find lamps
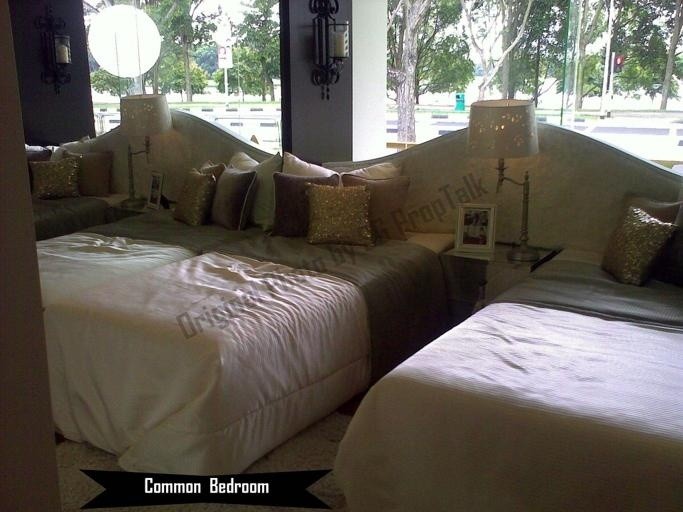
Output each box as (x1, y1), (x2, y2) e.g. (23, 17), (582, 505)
(467, 99), (540, 262)
(309, 0), (349, 100)
(120, 93), (172, 209)
(38, 3), (73, 97)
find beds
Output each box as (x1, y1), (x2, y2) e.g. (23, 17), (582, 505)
(43, 232), (457, 475)
(36, 208), (262, 310)
(32, 192), (128, 240)
(332, 248), (683, 512)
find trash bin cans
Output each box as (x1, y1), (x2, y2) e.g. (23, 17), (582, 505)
(456, 93), (464, 112)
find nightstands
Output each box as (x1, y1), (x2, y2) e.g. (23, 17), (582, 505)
(441, 242), (560, 326)
(105, 194), (151, 224)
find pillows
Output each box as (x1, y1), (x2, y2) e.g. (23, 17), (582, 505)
(601, 194), (683, 286)
(26, 138), (110, 199)
(269, 152), (410, 248)
(172, 151), (281, 233)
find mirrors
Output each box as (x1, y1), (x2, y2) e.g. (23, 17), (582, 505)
(8, 0), (293, 307)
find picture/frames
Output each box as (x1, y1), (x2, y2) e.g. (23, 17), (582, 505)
(146, 170), (164, 210)
(456, 202), (497, 256)
